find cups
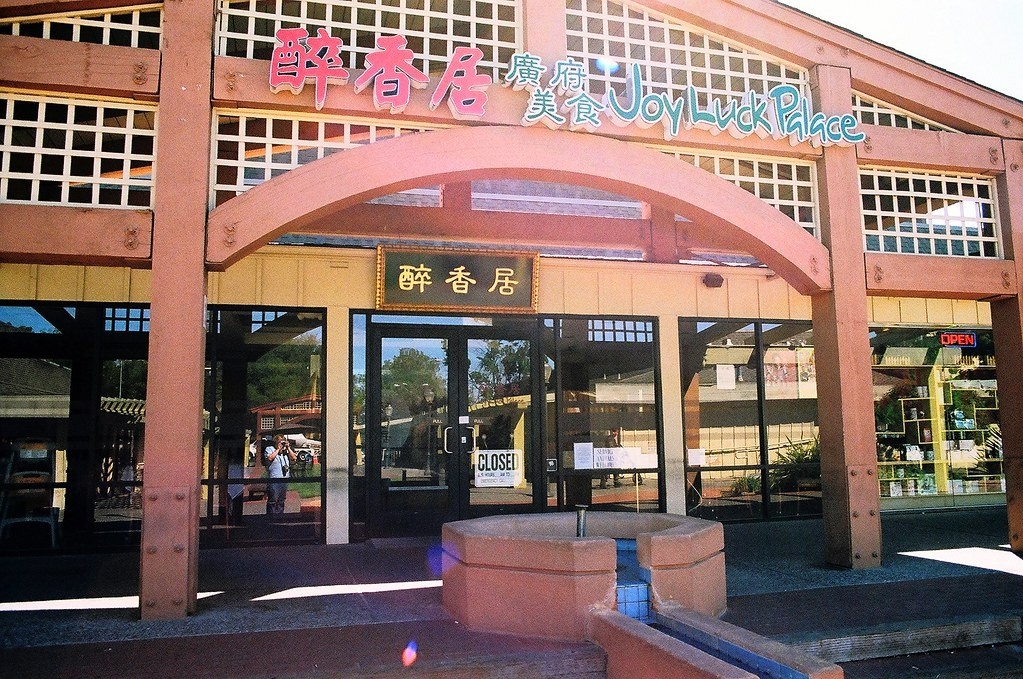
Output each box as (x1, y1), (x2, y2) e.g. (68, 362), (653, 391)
(911, 407), (918, 420)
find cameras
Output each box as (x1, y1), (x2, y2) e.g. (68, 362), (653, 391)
(281, 441), (286, 447)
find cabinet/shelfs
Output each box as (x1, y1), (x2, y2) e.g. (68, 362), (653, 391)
(871, 365), (1006, 498)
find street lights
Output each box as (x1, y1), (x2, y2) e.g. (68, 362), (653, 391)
(422, 387), (436, 476)
(384, 404), (394, 469)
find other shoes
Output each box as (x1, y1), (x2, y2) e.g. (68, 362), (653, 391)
(600, 485), (607, 489)
(615, 483), (621, 488)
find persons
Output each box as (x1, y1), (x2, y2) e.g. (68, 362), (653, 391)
(600, 426), (621, 488)
(264, 434), (297, 514)
